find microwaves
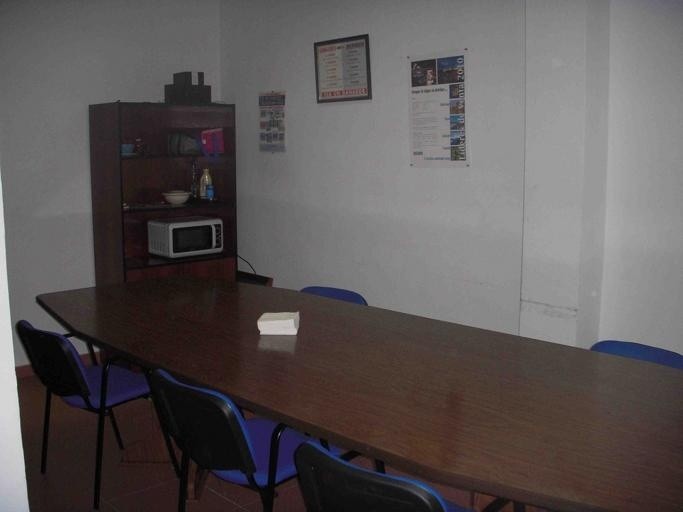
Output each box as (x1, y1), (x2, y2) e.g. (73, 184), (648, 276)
(148, 215), (223, 259)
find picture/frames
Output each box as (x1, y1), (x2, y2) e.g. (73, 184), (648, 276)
(313, 34), (372, 104)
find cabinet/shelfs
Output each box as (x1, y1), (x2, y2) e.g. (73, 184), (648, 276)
(88, 102), (238, 285)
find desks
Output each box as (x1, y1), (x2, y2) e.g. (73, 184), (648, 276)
(35, 272), (682, 511)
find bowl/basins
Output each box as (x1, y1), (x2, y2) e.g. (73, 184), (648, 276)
(161, 192), (192, 205)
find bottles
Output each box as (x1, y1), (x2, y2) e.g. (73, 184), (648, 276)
(190, 151), (214, 202)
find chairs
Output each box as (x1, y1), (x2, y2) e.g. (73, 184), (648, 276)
(146, 370), (389, 510)
(290, 440), (478, 512)
(16, 319), (182, 508)
